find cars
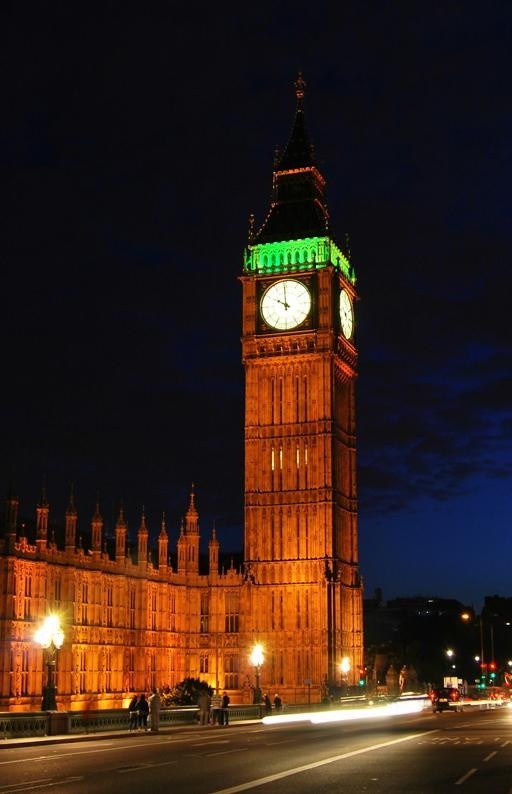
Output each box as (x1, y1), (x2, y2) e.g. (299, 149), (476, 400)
(430, 687), (464, 713)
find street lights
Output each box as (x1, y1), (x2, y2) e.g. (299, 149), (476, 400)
(33, 614), (65, 711)
(341, 657), (350, 697)
(250, 644), (264, 703)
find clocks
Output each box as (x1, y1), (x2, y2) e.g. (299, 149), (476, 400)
(259, 277), (312, 332)
(339, 287), (355, 342)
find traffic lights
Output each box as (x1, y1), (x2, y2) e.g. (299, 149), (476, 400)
(477, 663), (495, 689)
(358, 669), (366, 688)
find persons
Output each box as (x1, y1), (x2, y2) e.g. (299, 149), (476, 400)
(211, 693), (224, 725)
(221, 690), (229, 727)
(149, 687), (161, 731)
(264, 693), (272, 713)
(136, 694), (150, 732)
(128, 695), (139, 732)
(198, 690), (210, 727)
(273, 693), (283, 712)
